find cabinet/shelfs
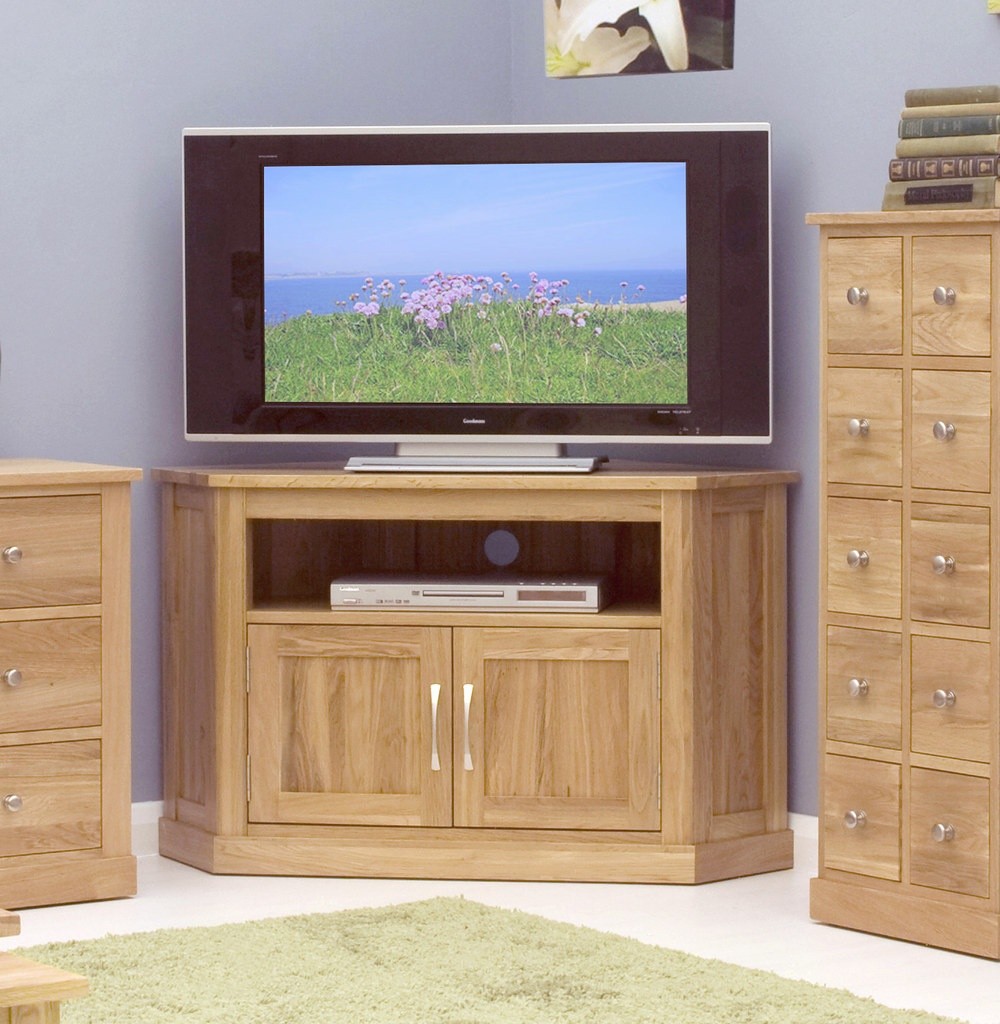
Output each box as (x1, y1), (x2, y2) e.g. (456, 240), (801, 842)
(2, 452), (145, 910)
(801, 208), (1000, 962)
(154, 464), (795, 889)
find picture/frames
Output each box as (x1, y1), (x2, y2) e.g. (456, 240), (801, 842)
(541, 0), (736, 83)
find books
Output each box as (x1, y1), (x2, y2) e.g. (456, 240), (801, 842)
(876, 85), (1000, 211)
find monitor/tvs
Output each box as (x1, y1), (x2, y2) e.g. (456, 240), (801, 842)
(180, 121), (772, 476)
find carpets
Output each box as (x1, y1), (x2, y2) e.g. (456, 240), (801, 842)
(0, 888), (976, 1023)
